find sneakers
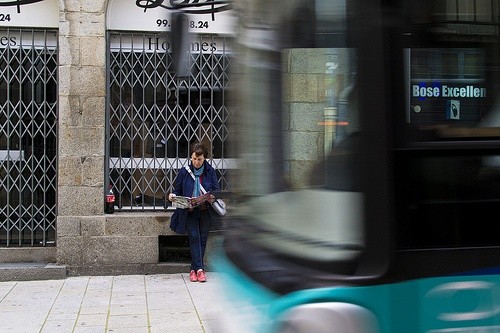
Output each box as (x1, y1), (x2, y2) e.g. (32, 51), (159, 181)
(190, 270), (197, 282)
(196, 270), (206, 281)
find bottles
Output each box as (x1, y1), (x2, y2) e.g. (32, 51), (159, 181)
(105, 185), (115, 214)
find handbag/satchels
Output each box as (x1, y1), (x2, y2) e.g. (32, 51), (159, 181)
(212, 198), (226, 216)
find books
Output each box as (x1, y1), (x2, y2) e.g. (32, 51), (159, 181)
(170, 191), (211, 210)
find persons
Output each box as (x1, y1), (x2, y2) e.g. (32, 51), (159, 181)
(168, 144), (220, 283)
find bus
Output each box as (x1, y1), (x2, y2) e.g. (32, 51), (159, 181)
(170, 1), (500, 333)
